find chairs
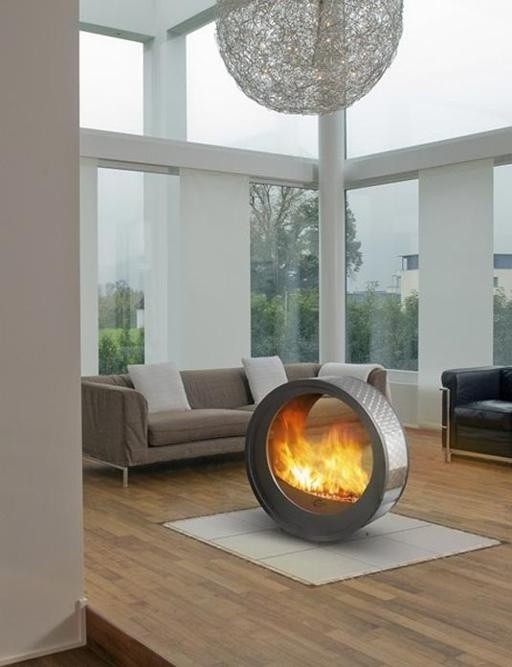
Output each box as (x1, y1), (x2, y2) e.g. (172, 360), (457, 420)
(442, 367), (511, 467)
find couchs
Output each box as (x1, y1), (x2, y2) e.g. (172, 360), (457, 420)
(81, 363), (389, 487)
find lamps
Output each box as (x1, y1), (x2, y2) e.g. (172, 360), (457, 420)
(217, 1), (404, 113)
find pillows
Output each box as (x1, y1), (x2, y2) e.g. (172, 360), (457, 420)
(242, 355), (288, 405)
(130, 364), (191, 414)
(154, 505), (508, 587)
